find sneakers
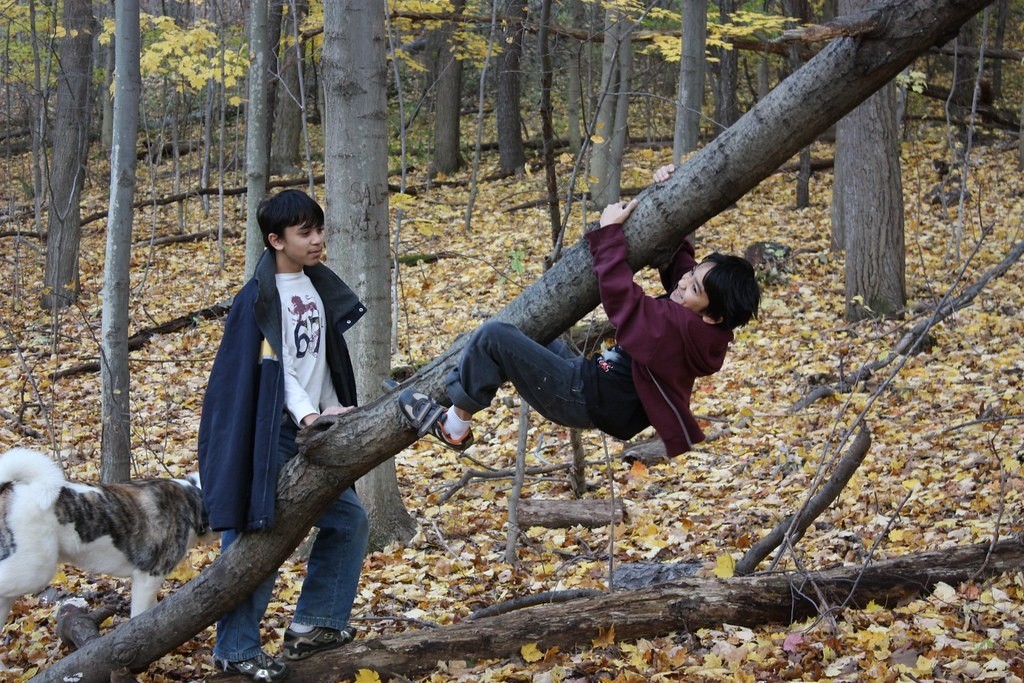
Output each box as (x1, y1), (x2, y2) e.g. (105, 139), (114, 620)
(215, 653), (290, 683)
(284, 623), (356, 661)
(398, 389), (474, 451)
(381, 378), (401, 395)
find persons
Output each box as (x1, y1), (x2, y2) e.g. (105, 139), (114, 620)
(379, 165), (761, 451)
(197, 189), (371, 683)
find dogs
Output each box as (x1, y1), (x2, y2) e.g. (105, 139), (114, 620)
(0, 449), (228, 628)
